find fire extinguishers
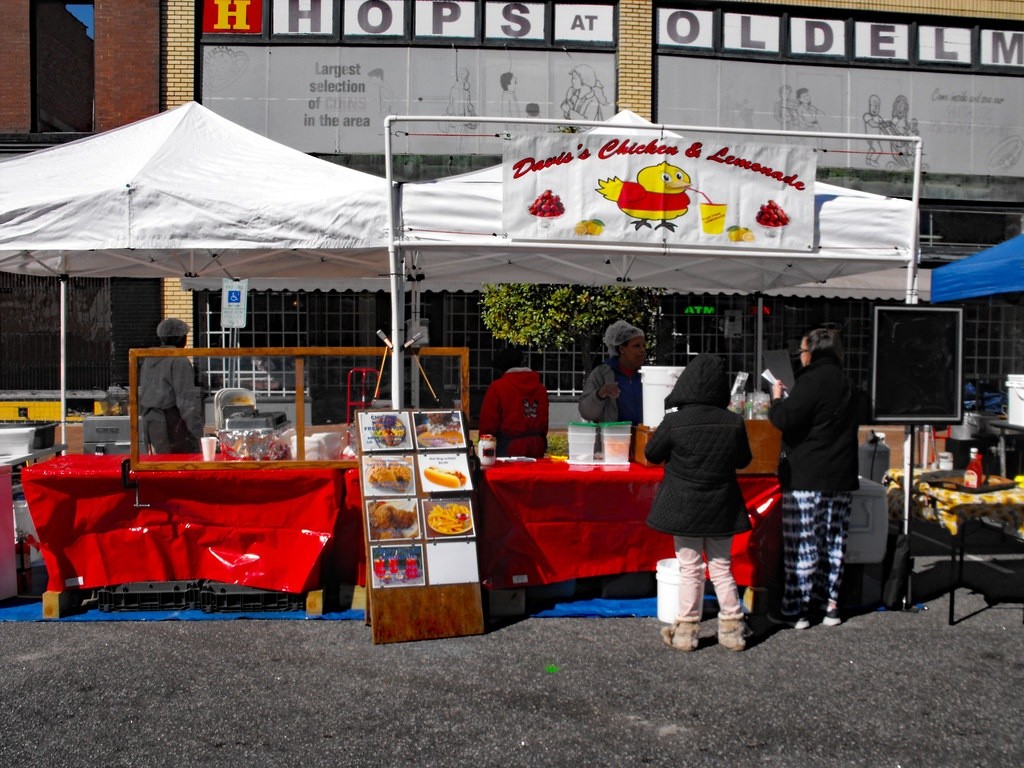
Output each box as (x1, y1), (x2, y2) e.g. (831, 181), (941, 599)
(14, 527), (40, 595)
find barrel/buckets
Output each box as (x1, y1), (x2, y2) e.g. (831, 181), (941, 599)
(939, 452), (953, 471)
(1005, 374), (1024, 427)
(656, 558), (706, 624)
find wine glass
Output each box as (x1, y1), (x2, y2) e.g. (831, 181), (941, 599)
(374, 556), (387, 586)
(405, 553), (417, 584)
(388, 555), (400, 583)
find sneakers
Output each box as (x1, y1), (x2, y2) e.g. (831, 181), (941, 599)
(809, 603), (842, 627)
(766, 609), (810, 629)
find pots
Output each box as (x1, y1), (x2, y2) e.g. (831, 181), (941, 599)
(950, 412), (981, 440)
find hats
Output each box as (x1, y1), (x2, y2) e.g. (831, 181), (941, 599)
(490, 347), (522, 372)
(157, 318), (189, 337)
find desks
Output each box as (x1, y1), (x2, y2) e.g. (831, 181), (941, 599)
(1, 442), (787, 619)
(882, 466), (1024, 626)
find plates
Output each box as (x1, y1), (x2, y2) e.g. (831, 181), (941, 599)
(428, 518), (472, 535)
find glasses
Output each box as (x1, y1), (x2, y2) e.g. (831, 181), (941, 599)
(798, 349), (809, 353)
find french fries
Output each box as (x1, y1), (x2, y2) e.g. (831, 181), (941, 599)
(429, 502), (472, 532)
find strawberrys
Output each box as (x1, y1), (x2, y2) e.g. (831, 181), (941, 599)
(756, 200), (789, 226)
(529, 190), (565, 217)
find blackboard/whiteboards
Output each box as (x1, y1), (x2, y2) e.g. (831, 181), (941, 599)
(867, 301), (965, 426)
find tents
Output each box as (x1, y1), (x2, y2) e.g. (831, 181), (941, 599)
(929, 233), (1024, 304)
(0, 107), (919, 607)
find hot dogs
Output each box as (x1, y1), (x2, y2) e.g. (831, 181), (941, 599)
(418, 430), (464, 446)
(424, 467), (466, 488)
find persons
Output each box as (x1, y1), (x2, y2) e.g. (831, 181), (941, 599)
(769, 328), (859, 629)
(578, 320), (649, 425)
(138, 319), (215, 453)
(475, 347), (550, 460)
(645, 356), (751, 652)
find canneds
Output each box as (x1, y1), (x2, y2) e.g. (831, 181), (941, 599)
(477, 435), (496, 465)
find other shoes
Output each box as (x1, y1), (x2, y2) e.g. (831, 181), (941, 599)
(717, 619), (754, 651)
(661, 620), (701, 651)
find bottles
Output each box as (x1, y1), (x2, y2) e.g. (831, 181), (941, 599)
(477, 435), (497, 465)
(963, 447), (983, 489)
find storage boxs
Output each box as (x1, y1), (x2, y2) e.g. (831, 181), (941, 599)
(566, 420), (635, 471)
(636, 424), (665, 466)
(1004, 373), (1024, 427)
(735, 419), (782, 475)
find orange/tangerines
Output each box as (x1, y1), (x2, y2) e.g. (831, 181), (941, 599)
(575, 220), (603, 235)
(728, 227), (755, 242)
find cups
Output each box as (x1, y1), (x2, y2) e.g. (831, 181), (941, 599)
(730, 390), (770, 420)
(200, 437), (217, 461)
